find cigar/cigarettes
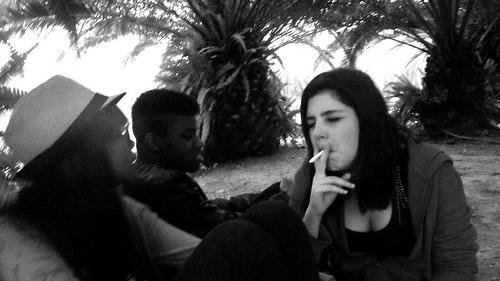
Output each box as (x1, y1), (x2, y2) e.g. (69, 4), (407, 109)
(309, 150), (325, 163)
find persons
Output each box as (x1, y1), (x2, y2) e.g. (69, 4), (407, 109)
(290, 69), (479, 281)
(120, 89), (297, 238)
(0, 76), (320, 281)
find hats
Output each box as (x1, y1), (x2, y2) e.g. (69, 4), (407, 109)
(3, 75), (126, 181)
